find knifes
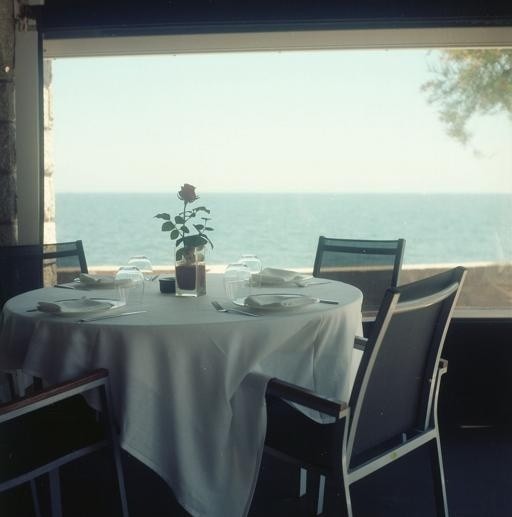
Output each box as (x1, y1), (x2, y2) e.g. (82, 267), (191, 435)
(54, 285), (90, 291)
(78, 310), (147, 323)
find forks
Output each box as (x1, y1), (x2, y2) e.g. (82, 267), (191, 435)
(211, 301), (261, 317)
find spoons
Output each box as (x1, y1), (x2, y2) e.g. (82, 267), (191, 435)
(294, 281), (330, 287)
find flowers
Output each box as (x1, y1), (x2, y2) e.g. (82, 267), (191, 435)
(152, 183), (215, 266)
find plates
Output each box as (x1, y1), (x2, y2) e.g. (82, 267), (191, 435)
(233, 293), (320, 312)
(50, 298), (126, 318)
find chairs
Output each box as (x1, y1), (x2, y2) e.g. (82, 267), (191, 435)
(0, 368), (129, 517)
(262, 265), (469, 517)
(313, 236), (406, 338)
(0, 240), (88, 401)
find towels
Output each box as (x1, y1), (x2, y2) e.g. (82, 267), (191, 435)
(80, 273), (115, 284)
(256, 267), (300, 284)
(244, 293), (309, 307)
(38, 295), (114, 314)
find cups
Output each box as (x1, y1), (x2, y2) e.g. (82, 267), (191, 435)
(223, 254), (263, 299)
(114, 255), (152, 317)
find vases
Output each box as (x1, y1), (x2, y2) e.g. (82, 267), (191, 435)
(175, 246), (206, 297)
